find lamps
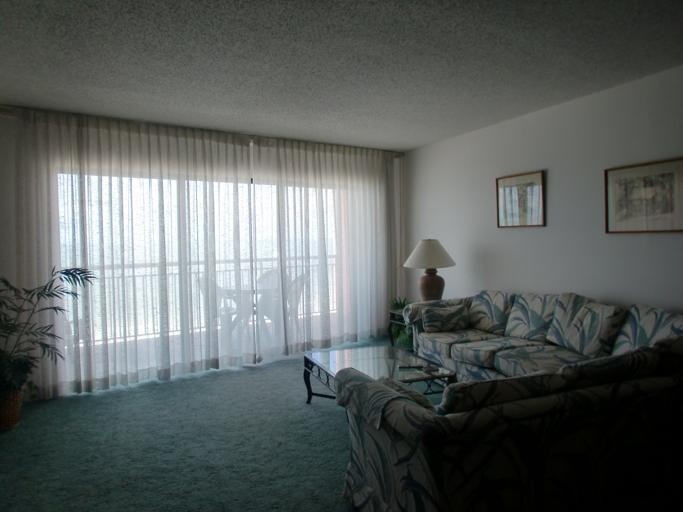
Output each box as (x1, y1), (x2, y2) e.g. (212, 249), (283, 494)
(401, 239), (455, 301)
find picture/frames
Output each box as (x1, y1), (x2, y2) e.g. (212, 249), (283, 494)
(604, 156), (683, 234)
(495, 169), (546, 228)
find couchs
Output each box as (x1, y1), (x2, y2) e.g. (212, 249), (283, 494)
(333, 289), (682, 512)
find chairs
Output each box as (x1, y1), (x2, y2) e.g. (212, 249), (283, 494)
(191, 262), (312, 354)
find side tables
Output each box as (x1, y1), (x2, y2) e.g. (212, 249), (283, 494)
(386, 309), (406, 346)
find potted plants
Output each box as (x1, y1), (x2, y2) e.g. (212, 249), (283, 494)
(0, 265), (99, 430)
